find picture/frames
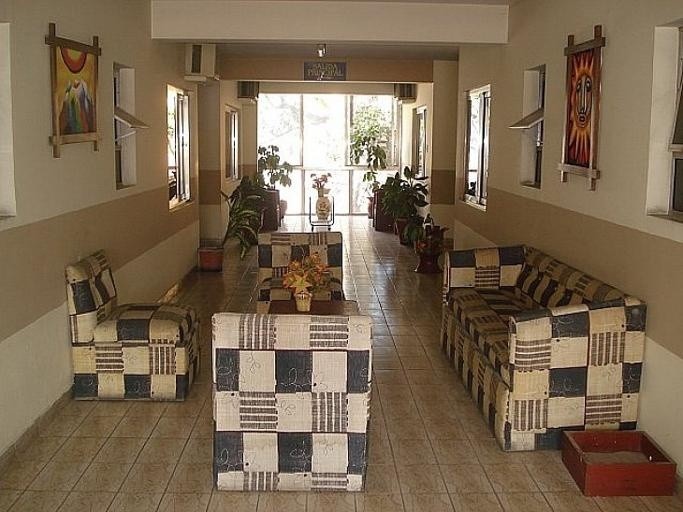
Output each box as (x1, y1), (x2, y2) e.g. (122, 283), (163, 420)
(558, 25), (605, 191)
(44, 23), (102, 158)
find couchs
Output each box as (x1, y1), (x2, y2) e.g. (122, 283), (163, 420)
(440, 244), (646, 454)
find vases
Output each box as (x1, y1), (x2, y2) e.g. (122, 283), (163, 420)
(294, 293), (312, 312)
(316, 197), (331, 219)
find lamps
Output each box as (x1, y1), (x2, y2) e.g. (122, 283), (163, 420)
(317, 44), (327, 58)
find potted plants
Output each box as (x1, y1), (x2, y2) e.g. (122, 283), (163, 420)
(403, 214), (450, 274)
(349, 104), (386, 218)
(198, 176), (269, 271)
(258, 144), (293, 218)
(382, 166), (429, 246)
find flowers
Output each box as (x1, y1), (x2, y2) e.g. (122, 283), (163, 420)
(311, 173), (332, 197)
(283, 252), (328, 293)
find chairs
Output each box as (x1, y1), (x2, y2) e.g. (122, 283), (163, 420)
(211, 312), (373, 493)
(65, 249), (200, 402)
(258, 233), (343, 315)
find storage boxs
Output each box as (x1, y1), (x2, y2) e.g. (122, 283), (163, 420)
(562, 429), (677, 496)
